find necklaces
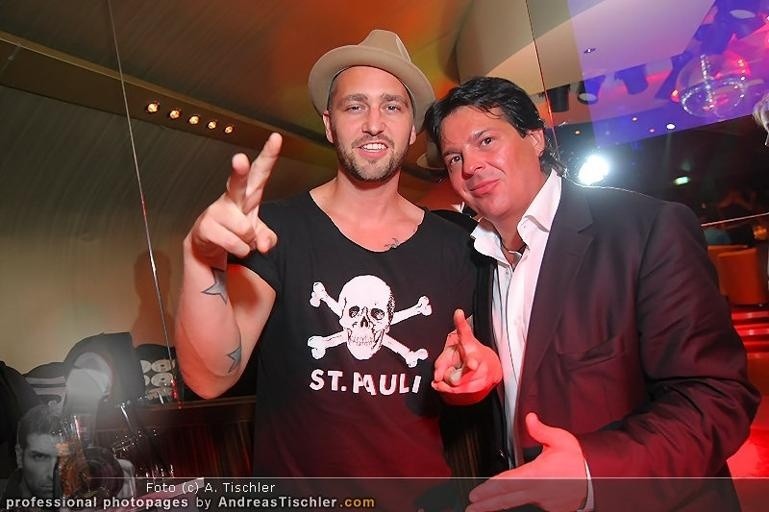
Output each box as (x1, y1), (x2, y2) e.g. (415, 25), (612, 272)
(500, 238), (527, 263)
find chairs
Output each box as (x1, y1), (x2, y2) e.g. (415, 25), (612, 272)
(707, 244), (769, 310)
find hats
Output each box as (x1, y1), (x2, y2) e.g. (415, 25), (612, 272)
(308, 30), (434, 135)
(417, 139), (446, 172)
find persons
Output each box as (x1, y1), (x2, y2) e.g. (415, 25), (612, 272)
(0, 403), (71, 512)
(172, 28), (503, 511)
(421, 74), (763, 512)
